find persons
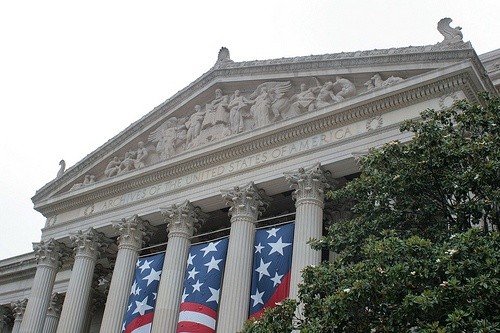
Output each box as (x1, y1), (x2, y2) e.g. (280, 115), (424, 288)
(63, 65), (401, 189)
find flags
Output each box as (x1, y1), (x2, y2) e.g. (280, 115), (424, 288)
(175, 238), (229, 333)
(121, 253), (165, 333)
(248, 223), (293, 319)
